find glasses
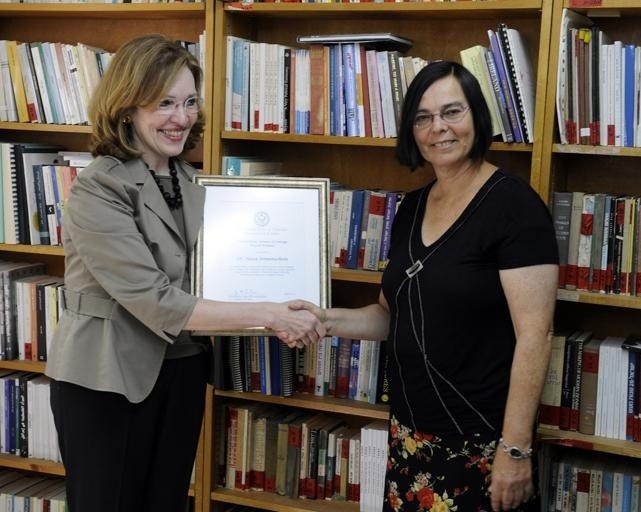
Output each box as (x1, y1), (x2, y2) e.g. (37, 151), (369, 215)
(155, 95), (205, 115)
(414, 104), (470, 130)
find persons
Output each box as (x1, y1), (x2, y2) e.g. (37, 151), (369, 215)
(47, 33), (327, 512)
(264, 61), (560, 512)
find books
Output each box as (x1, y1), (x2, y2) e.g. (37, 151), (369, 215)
(230, 335), (389, 406)
(221, 155), (405, 273)
(0, 467), (68, 512)
(555, 9), (641, 148)
(0, 142), (94, 246)
(226, 24), (535, 143)
(550, 453), (641, 512)
(0, 40), (116, 127)
(538, 330), (641, 441)
(552, 191), (641, 298)
(0, 368), (63, 463)
(219, 402), (389, 512)
(0, 261), (66, 362)
(176, 30), (205, 103)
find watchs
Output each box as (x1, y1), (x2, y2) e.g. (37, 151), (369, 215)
(500, 438), (533, 460)
